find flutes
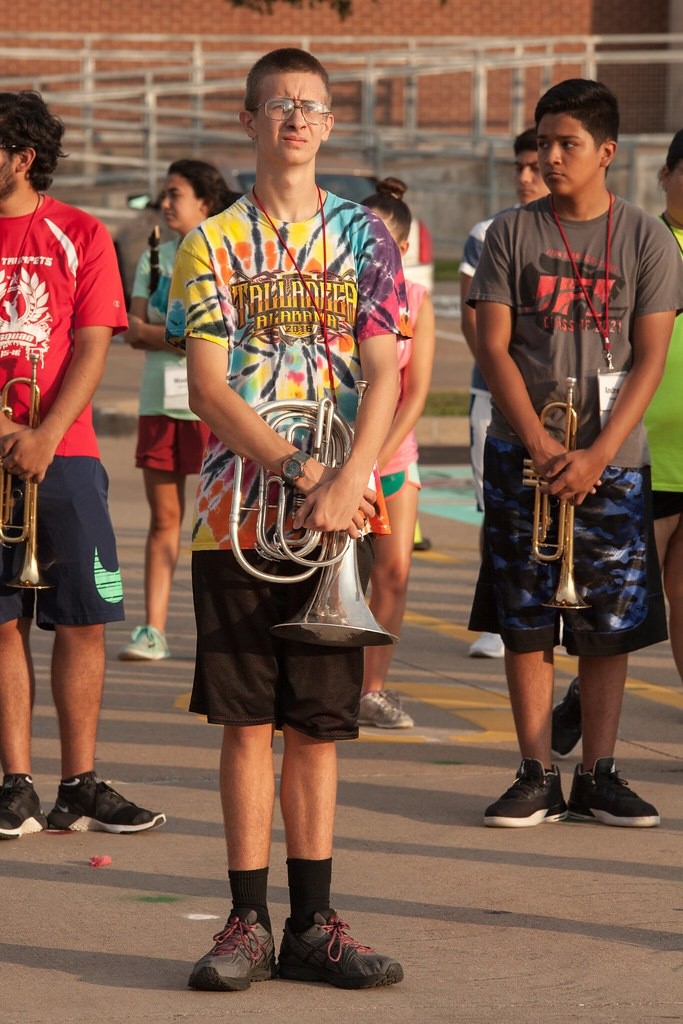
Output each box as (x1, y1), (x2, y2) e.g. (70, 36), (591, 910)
(148, 224), (160, 294)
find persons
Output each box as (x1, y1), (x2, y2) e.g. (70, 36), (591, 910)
(0, 90), (166, 839)
(119, 158), (235, 661)
(350, 178), (435, 729)
(459, 78), (683, 826)
(164, 46), (412, 988)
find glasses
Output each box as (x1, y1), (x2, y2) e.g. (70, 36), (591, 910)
(253, 98), (331, 125)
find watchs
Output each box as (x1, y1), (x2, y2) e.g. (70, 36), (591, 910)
(278, 450), (312, 487)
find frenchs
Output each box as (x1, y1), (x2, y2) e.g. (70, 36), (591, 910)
(220, 379), (402, 652)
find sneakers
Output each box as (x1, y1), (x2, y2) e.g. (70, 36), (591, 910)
(277, 907), (404, 989)
(484, 758), (569, 827)
(567, 755), (660, 827)
(357, 690), (415, 728)
(47, 777), (166, 834)
(469, 633), (505, 658)
(117, 625), (168, 661)
(0, 775), (48, 837)
(188, 908), (276, 991)
(550, 676), (584, 758)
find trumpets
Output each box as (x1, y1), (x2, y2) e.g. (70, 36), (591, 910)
(519, 373), (593, 612)
(0, 351), (53, 594)
